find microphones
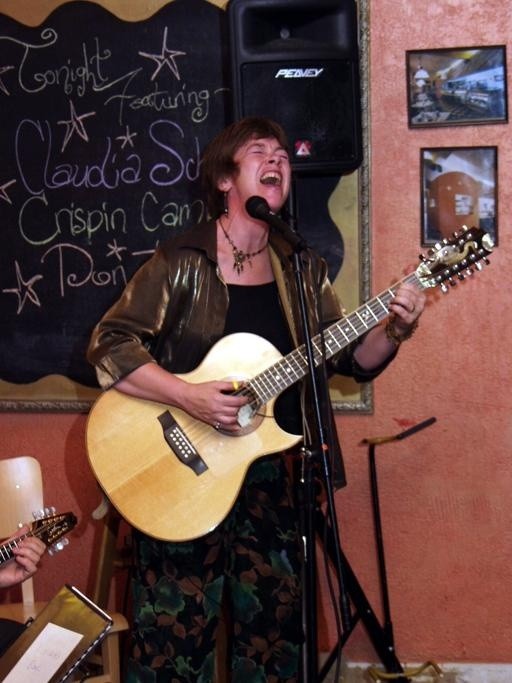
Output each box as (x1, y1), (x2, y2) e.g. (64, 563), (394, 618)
(245, 196), (308, 252)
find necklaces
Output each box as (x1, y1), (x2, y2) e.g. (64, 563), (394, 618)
(218, 217), (269, 275)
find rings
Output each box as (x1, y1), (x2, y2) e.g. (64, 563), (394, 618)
(411, 304), (415, 313)
(215, 421), (221, 429)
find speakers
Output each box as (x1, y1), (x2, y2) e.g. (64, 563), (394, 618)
(225, 0), (364, 175)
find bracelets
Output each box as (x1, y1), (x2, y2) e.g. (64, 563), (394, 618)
(385, 313), (419, 344)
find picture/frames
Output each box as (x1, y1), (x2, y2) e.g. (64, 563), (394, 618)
(405, 45), (509, 250)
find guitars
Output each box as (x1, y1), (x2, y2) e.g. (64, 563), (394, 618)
(0, 506), (77, 564)
(85, 226), (497, 547)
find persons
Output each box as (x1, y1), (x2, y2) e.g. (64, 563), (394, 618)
(0, 526), (47, 589)
(85, 116), (426, 683)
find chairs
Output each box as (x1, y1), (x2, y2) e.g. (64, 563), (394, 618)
(2, 454), (130, 682)
